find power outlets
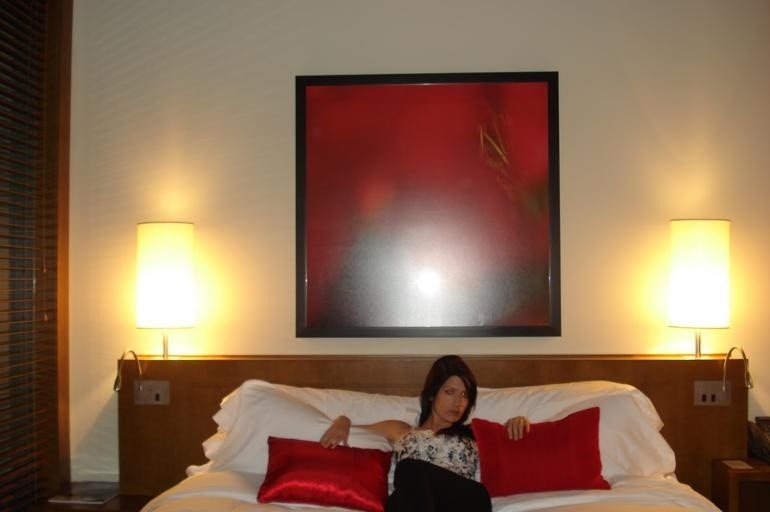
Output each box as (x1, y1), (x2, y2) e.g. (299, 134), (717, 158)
(693, 381), (730, 405)
(134, 380), (169, 405)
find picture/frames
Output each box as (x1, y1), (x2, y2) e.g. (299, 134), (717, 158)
(296, 71), (562, 336)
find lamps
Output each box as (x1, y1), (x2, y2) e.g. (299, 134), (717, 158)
(669, 217), (732, 357)
(137, 222), (198, 359)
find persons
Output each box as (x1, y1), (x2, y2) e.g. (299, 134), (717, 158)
(319, 355), (530, 511)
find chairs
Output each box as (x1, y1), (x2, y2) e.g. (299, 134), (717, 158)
(750, 416), (770, 460)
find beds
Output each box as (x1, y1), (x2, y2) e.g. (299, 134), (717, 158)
(112, 355), (751, 511)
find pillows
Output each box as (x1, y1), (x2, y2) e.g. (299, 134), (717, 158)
(257, 437), (394, 510)
(473, 406), (613, 495)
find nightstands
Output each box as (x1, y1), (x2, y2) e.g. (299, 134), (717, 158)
(711, 459), (769, 511)
